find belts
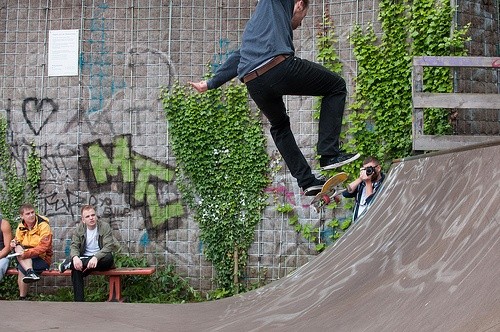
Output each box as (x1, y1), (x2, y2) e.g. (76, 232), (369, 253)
(244, 54), (290, 85)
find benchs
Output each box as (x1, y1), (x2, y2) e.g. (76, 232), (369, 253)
(6, 267), (156, 302)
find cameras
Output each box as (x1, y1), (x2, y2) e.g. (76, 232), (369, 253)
(364, 166), (378, 183)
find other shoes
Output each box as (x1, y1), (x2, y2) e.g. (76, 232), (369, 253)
(303, 179), (326, 196)
(58, 259), (73, 273)
(320, 141), (361, 171)
(22, 268), (40, 284)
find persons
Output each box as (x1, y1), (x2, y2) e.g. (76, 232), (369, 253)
(9, 204), (53, 299)
(190, 0), (361, 196)
(59, 205), (116, 302)
(341, 156), (386, 223)
(0, 216), (13, 285)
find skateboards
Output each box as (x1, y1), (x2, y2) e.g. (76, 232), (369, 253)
(311, 172), (349, 214)
